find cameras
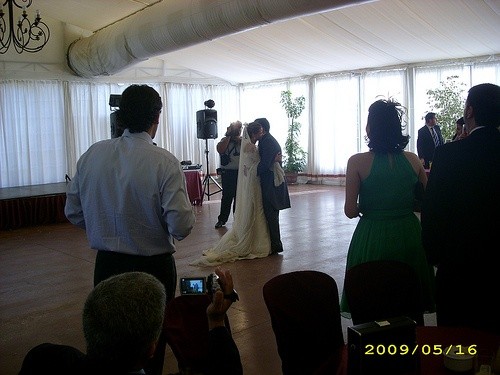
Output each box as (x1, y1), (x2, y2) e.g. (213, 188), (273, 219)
(180, 273), (223, 302)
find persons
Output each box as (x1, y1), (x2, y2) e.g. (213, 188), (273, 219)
(213, 121), (241, 230)
(64, 85), (194, 375)
(422, 83), (500, 334)
(452, 116), (468, 142)
(18, 268), (245, 375)
(199, 121), (285, 264)
(417, 112), (444, 168)
(340, 100), (431, 323)
(244, 118), (291, 258)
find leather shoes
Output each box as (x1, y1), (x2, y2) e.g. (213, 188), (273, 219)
(215, 221), (226, 228)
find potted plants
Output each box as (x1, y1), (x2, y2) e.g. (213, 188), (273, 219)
(279, 89), (310, 184)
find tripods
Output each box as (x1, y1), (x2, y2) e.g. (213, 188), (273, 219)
(201, 139), (222, 201)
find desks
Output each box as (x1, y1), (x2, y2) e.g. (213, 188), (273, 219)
(334, 325), (500, 375)
(182, 169), (202, 206)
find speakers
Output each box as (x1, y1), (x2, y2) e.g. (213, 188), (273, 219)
(196, 110), (217, 140)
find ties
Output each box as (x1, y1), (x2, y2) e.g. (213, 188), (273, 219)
(430, 128), (440, 147)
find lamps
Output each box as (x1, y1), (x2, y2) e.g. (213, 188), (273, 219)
(0, 0), (51, 54)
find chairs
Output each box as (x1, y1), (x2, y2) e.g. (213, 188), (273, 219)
(162, 294), (244, 375)
(262, 270), (344, 375)
(344, 260), (434, 326)
